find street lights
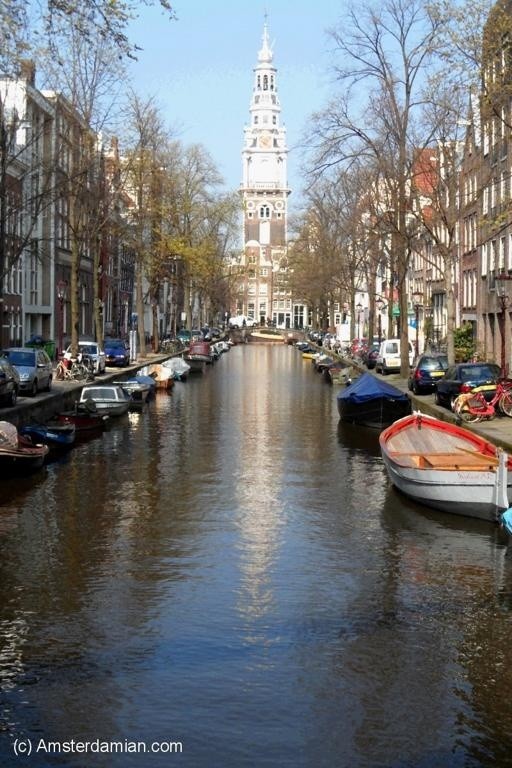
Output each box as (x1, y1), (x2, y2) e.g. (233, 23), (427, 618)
(489, 271), (512, 366)
(55, 277), (69, 359)
(376, 297), (385, 340)
(356, 301), (365, 338)
(167, 249), (182, 336)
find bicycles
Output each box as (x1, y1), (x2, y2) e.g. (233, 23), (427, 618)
(54, 345), (95, 383)
(449, 375), (512, 424)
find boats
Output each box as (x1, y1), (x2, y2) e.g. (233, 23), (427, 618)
(335, 370), (415, 438)
(134, 361), (176, 386)
(283, 337), (355, 386)
(186, 337), (235, 366)
(376, 408), (512, 524)
(160, 355), (191, 382)
(501, 506), (512, 539)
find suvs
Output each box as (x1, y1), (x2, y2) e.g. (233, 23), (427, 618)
(102, 337), (130, 352)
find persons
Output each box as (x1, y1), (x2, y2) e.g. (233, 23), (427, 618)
(242, 318), (246, 327)
(267, 315), (272, 326)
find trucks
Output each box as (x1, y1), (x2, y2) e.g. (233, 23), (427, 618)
(228, 314), (258, 329)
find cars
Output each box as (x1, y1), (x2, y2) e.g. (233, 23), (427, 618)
(103, 346), (130, 367)
(431, 359), (506, 413)
(302, 323), (388, 366)
(3, 345), (54, 398)
(159, 324), (222, 351)
(0, 347), (21, 409)
(59, 340), (107, 375)
(0, 374), (158, 471)
(406, 350), (450, 396)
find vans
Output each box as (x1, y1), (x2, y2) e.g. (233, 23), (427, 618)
(374, 336), (416, 375)
